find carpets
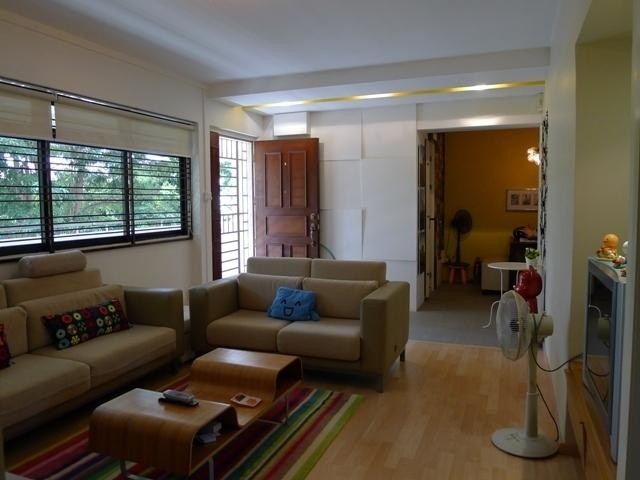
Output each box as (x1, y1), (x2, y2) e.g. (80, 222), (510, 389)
(5, 369), (363, 480)
(408, 282), (542, 351)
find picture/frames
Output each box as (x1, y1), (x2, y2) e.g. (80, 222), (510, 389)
(505, 188), (538, 213)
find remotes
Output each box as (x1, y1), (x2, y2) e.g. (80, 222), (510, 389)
(159, 398), (199, 406)
(164, 389), (194, 402)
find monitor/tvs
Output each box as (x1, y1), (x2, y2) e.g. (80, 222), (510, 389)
(582, 258), (627, 462)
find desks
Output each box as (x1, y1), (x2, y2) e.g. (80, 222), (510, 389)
(483, 262), (537, 329)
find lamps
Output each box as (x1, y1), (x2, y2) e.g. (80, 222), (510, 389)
(51, 100), (57, 140)
(527, 146), (542, 167)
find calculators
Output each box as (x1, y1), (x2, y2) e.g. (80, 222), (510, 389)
(230, 392), (263, 406)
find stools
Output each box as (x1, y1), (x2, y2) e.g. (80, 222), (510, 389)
(448, 266), (468, 289)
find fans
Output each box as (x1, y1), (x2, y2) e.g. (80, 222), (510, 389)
(490, 290), (560, 459)
(587, 303), (612, 349)
(449, 209), (472, 268)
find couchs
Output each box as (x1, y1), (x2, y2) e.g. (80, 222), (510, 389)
(0, 268), (185, 442)
(187, 255), (410, 393)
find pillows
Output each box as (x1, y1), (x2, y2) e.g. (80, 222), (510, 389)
(302, 277), (379, 320)
(16, 284), (128, 352)
(39, 297), (132, 351)
(0, 324), (15, 369)
(267, 287), (321, 321)
(238, 273), (302, 312)
(0, 306), (29, 358)
(17, 250), (87, 278)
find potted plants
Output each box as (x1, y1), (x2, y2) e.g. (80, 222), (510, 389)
(525, 248), (540, 269)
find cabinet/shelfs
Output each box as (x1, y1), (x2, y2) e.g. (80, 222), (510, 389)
(509, 242), (537, 291)
(481, 257), (510, 292)
